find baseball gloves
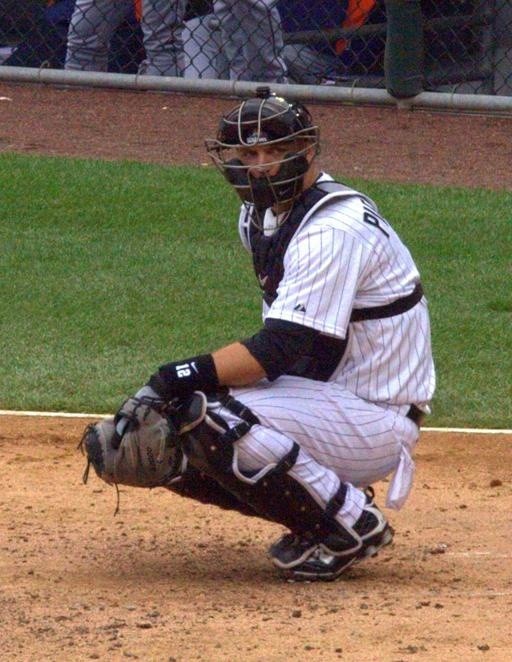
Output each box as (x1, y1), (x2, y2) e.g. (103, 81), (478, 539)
(77, 397), (182, 515)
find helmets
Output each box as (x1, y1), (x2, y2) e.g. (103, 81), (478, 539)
(203, 85), (321, 213)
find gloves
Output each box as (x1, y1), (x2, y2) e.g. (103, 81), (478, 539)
(109, 374), (171, 451)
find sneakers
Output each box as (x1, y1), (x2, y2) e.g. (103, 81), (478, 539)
(269, 485), (396, 580)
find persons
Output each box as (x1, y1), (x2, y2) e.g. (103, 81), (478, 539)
(64, 0), (184, 78)
(180, 1), (292, 85)
(70, 85), (437, 583)
